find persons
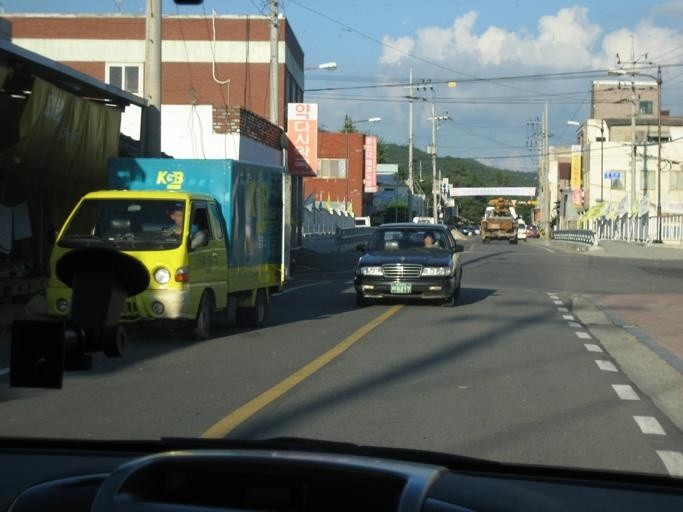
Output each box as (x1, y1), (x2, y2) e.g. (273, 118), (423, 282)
(423, 231), (443, 249)
(159, 204), (207, 253)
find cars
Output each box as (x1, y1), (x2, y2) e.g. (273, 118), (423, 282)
(458, 225), (479, 235)
(351, 221), (465, 308)
(525, 225), (541, 239)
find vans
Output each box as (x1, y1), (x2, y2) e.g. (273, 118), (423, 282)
(516, 219), (528, 242)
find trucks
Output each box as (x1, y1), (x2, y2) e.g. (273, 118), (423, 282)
(479, 198), (520, 245)
(40, 151), (294, 342)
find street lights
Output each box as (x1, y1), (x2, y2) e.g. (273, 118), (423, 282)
(567, 117), (606, 205)
(345, 116), (383, 213)
(605, 64), (665, 244)
